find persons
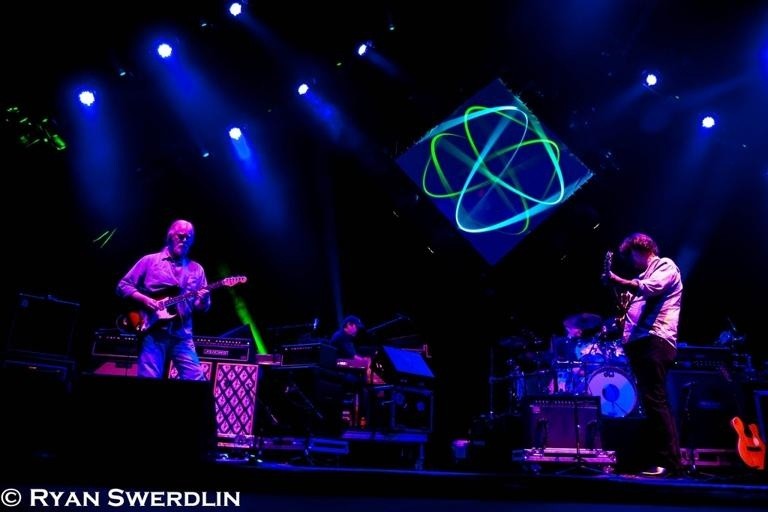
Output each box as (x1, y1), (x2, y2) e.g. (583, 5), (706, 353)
(549, 318), (584, 362)
(320, 316), (372, 373)
(113, 218), (214, 382)
(600, 233), (685, 477)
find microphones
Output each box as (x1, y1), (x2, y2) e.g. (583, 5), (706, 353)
(536, 414), (549, 426)
(313, 318), (317, 329)
(586, 421), (599, 430)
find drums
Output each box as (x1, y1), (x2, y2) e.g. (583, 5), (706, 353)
(586, 369), (638, 419)
(549, 362), (586, 394)
(576, 338), (603, 364)
(606, 339), (630, 366)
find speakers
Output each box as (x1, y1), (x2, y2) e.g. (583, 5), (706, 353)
(69, 374), (210, 488)
(522, 395), (604, 449)
(253, 366), (342, 437)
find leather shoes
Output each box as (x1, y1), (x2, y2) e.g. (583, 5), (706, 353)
(640, 464), (683, 477)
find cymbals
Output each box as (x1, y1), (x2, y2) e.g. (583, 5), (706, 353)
(564, 314), (602, 330)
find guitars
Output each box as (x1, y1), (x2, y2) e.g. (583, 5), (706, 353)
(731, 368), (765, 471)
(122, 275), (248, 333)
(602, 251), (633, 327)
